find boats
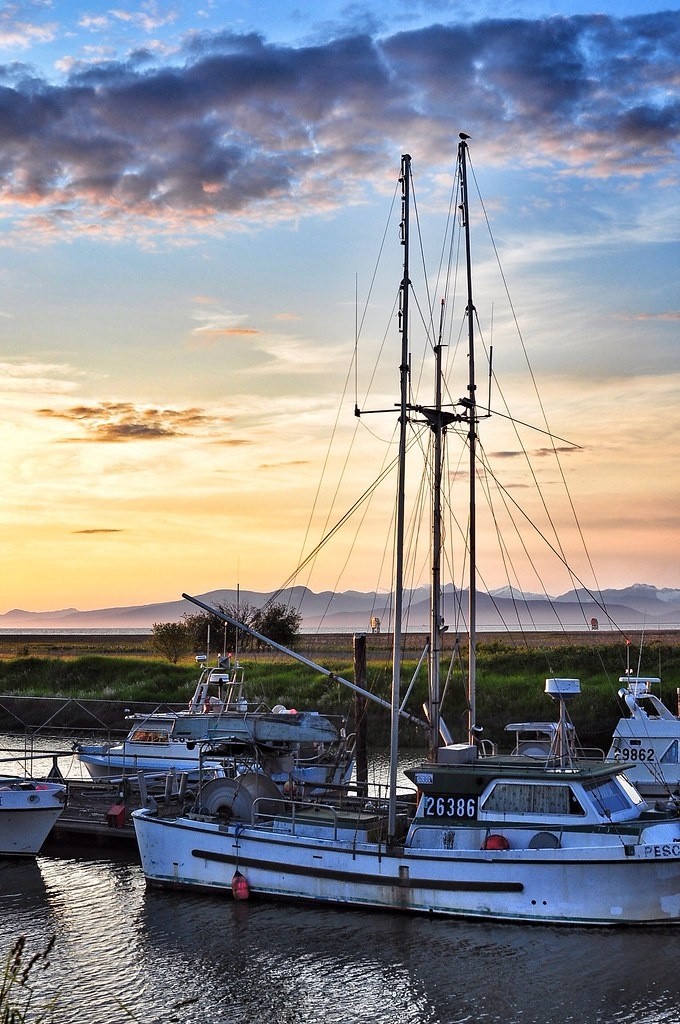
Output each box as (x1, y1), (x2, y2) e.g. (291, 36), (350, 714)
(0, 589), (680, 861)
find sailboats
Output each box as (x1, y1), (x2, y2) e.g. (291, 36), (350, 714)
(131, 130), (680, 930)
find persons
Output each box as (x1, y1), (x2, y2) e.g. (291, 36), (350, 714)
(188, 687), (223, 713)
